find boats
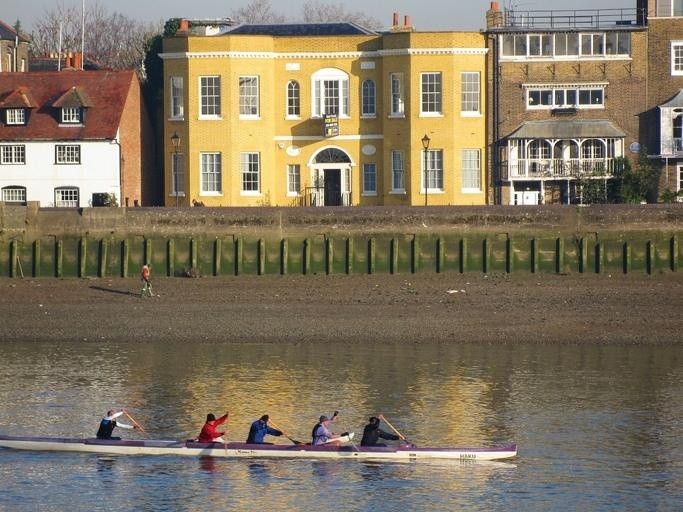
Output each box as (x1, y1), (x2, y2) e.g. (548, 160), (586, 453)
(0, 434), (517, 461)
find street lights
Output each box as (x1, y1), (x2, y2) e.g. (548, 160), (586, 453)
(170, 130), (181, 208)
(421, 134), (431, 206)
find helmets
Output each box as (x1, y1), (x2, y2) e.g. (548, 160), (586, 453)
(207, 413), (216, 421)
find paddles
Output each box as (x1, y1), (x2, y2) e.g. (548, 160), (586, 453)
(382, 417), (417, 448)
(269, 420), (302, 445)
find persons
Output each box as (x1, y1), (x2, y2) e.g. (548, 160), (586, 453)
(361, 414), (402, 446)
(141, 264), (155, 298)
(97, 411), (136, 440)
(193, 200), (201, 208)
(563, 189), (568, 204)
(312, 411), (349, 446)
(247, 415), (282, 444)
(199, 414), (228, 443)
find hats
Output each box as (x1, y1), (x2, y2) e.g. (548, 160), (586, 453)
(319, 415), (329, 423)
